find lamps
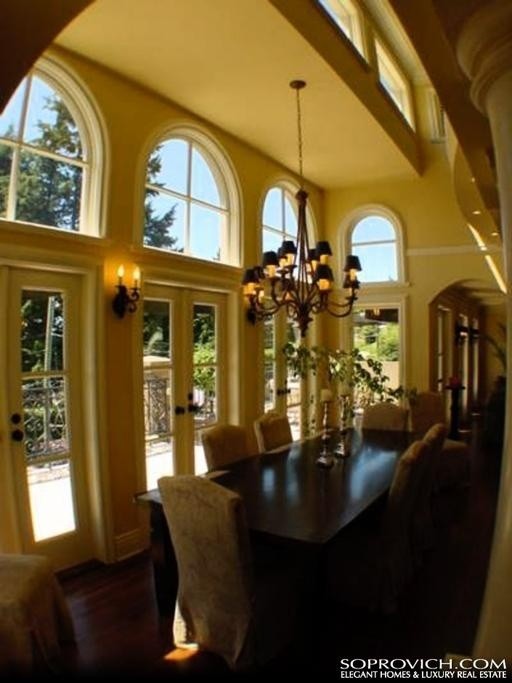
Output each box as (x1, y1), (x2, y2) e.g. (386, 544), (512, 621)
(237, 78), (361, 337)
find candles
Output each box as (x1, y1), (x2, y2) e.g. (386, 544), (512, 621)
(117, 263), (142, 288)
